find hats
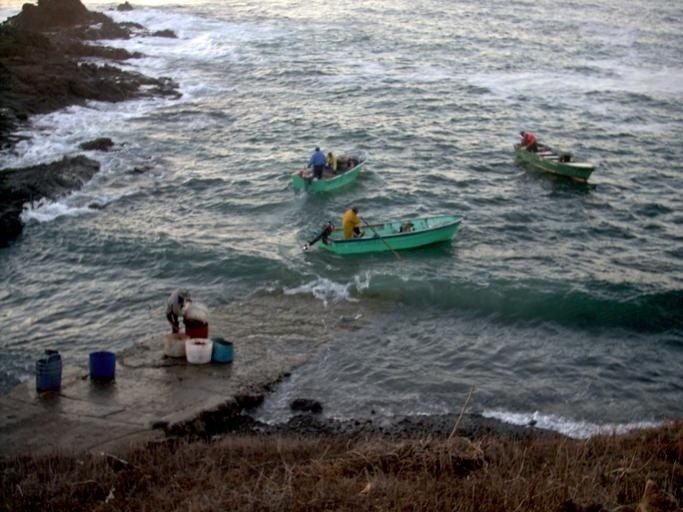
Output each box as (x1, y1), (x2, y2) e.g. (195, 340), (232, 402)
(178, 288), (191, 300)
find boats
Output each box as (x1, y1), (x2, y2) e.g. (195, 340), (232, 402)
(290, 149), (369, 192)
(303, 214), (465, 255)
(513, 138), (596, 183)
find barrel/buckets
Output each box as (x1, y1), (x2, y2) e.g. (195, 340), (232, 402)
(185, 339), (213, 364)
(36, 354), (62, 392)
(213, 337), (234, 362)
(163, 334), (189, 357)
(90, 351), (115, 385)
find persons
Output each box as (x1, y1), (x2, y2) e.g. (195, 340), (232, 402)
(306, 146), (326, 179)
(342, 207), (364, 239)
(518, 131), (537, 153)
(164, 286), (191, 334)
(324, 152), (337, 174)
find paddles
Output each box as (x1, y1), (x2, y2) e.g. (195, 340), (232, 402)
(360, 216), (403, 261)
